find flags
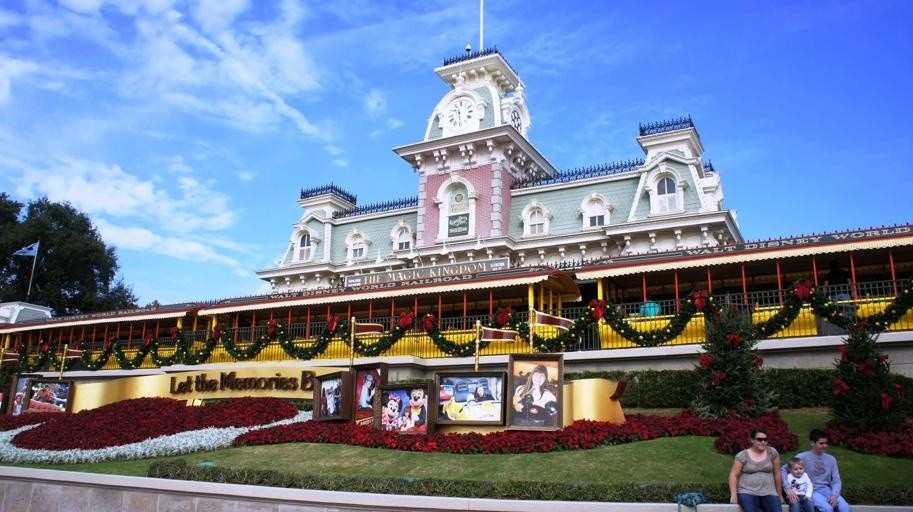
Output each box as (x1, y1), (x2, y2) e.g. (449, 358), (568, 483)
(11, 241), (39, 257)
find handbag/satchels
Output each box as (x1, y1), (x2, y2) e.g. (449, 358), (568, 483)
(674, 493), (704, 506)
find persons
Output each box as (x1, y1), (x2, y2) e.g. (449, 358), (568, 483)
(438, 377), (454, 386)
(473, 382), (495, 403)
(0, 389), (3, 408)
(395, 406), (415, 432)
(782, 457), (814, 512)
(30, 383), (68, 406)
(510, 363), (556, 427)
(12, 393), (23, 416)
(727, 427), (785, 511)
(320, 381), (342, 418)
(358, 375), (375, 409)
(779, 428), (851, 512)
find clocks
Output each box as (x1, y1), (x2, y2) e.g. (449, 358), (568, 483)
(447, 99), (476, 130)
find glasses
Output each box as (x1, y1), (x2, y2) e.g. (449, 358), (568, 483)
(754, 438), (768, 441)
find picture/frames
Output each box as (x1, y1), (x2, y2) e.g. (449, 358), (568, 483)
(8, 374), (75, 416)
(312, 353), (565, 436)
